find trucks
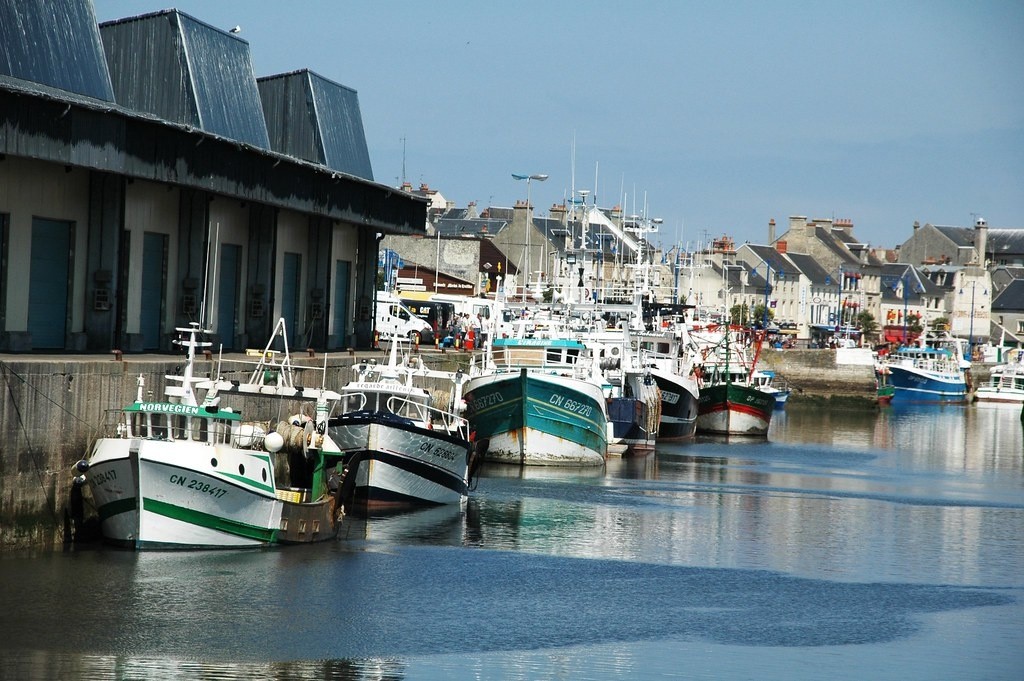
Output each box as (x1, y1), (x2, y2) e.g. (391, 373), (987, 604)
(433, 294), (500, 341)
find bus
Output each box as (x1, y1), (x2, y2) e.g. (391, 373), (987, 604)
(402, 297), (455, 340)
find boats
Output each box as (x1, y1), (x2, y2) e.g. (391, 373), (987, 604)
(972, 346), (1024, 403)
(287, 227), (489, 514)
(453, 133), (791, 466)
(872, 342), (973, 401)
(70, 218), (346, 552)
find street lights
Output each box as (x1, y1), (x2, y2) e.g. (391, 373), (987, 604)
(890, 272), (921, 346)
(593, 233), (618, 303)
(511, 172), (548, 314)
(823, 261), (858, 336)
(957, 279), (989, 353)
(752, 253), (784, 329)
(661, 243), (695, 305)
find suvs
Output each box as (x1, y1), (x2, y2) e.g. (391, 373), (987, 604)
(371, 296), (434, 344)
